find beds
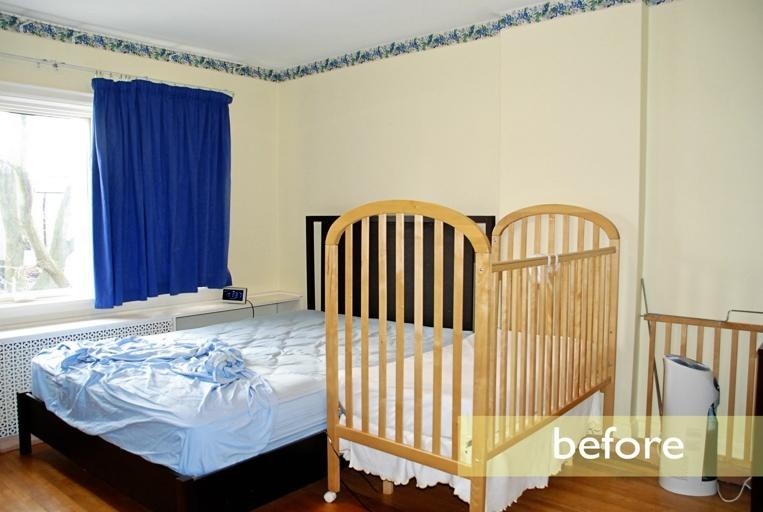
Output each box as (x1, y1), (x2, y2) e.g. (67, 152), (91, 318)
(324, 201), (621, 511)
(16, 216), (496, 512)
